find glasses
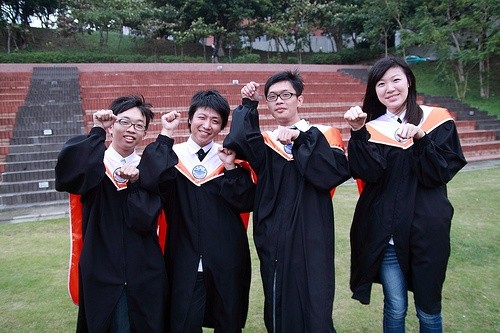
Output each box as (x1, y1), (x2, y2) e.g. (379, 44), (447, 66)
(114, 119), (146, 131)
(266, 91), (295, 101)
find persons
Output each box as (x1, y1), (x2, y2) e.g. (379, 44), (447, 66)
(224, 70), (352, 333)
(138, 89), (257, 333)
(344, 57), (468, 333)
(55, 93), (168, 333)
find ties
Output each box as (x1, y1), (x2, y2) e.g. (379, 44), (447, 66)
(398, 118), (401, 123)
(196, 149), (206, 162)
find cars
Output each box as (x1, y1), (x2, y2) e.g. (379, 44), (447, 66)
(405, 55), (430, 63)
